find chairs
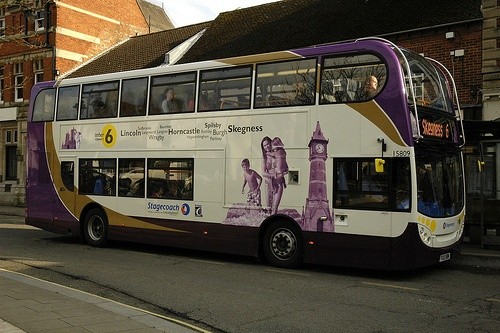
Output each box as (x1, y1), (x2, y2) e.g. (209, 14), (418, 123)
(220, 100), (238, 109)
(356, 165), (374, 190)
(168, 98), (184, 112)
(335, 80), (358, 102)
(269, 95), (283, 105)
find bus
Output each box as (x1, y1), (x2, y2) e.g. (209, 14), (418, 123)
(24, 37), (466, 272)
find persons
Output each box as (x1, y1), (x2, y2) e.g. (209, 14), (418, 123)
(354, 74), (380, 100)
(94, 160), (194, 198)
(88, 86), (222, 117)
(261, 135), (288, 215)
(241, 157), (262, 206)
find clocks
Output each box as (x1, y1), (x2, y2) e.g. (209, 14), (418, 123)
(315, 143), (325, 154)
